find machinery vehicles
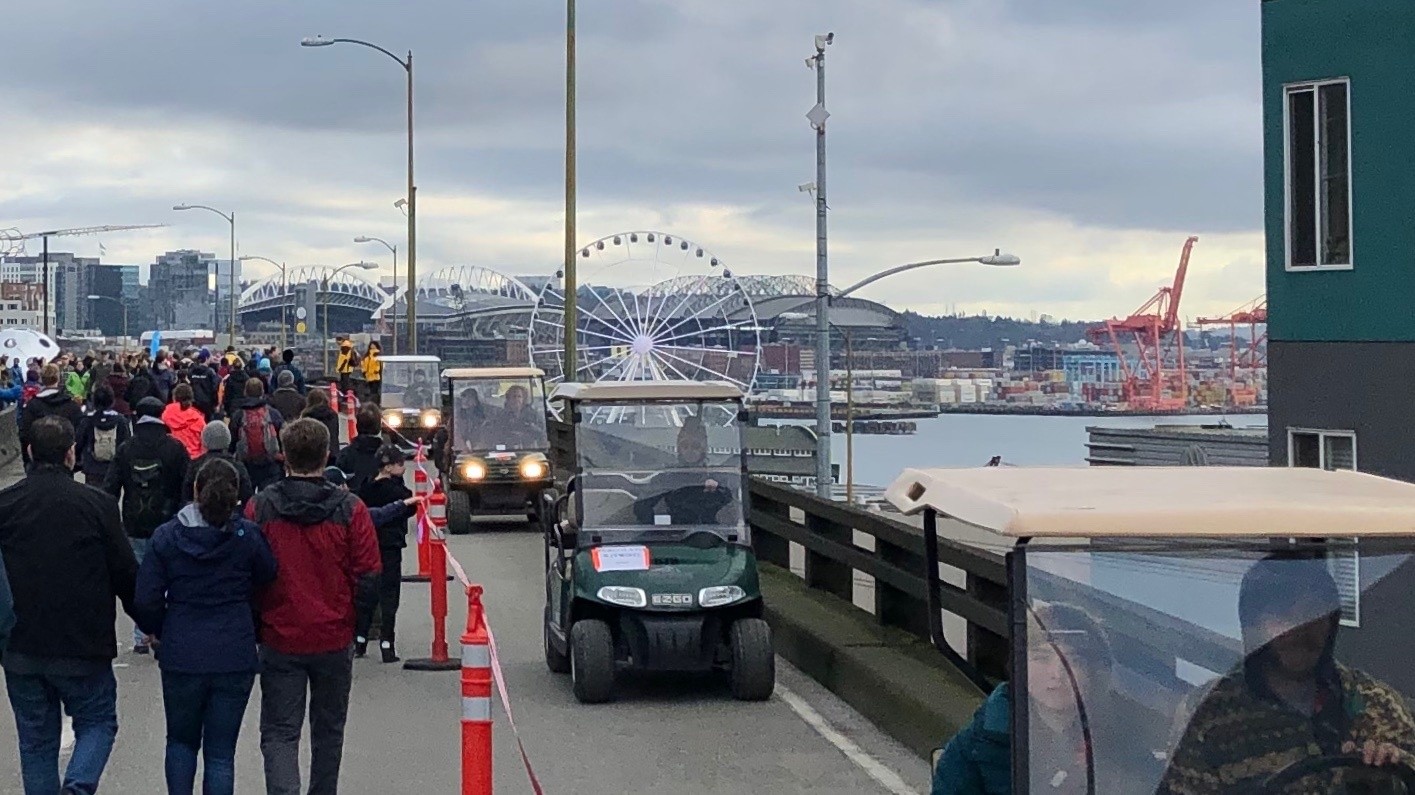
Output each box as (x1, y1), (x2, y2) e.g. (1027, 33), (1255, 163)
(451, 284), (483, 367)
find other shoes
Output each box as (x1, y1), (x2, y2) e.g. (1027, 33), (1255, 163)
(355, 633), (369, 657)
(380, 640), (400, 663)
(133, 645), (150, 654)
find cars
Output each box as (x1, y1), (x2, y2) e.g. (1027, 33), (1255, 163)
(291, 339), (339, 357)
(1104, 403), (1118, 412)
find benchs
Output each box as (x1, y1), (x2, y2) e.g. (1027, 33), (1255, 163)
(380, 392), (436, 408)
(556, 472), (740, 549)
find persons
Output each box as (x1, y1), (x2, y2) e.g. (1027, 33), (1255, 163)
(403, 370), (433, 406)
(1169, 553), (1415, 795)
(0, 417), (141, 795)
(932, 603), (1163, 795)
(448, 386), (543, 447)
(335, 337), (355, 402)
(245, 418), (381, 795)
(360, 341), (382, 395)
(634, 416), (732, 524)
(133, 458), (277, 795)
(0, 345), (423, 662)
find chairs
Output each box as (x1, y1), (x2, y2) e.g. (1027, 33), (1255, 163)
(568, 489), (639, 528)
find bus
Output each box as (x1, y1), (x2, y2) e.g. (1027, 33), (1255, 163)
(140, 329), (214, 349)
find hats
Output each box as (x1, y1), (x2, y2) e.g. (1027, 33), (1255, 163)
(322, 466), (356, 483)
(201, 419), (231, 450)
(27, 370), (40, 381)
(1027, 604), (1114, 707)
(677, 415), (708, 451)
(372, 443), (415, 468)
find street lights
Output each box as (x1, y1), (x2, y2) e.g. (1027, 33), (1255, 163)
(173, 202), (235, 346)
(324, 260), (378, 375)
(913, 338), (921, 377)
(300, 35), (417, 387)
(1027, 338), (1034, 380)
(88, 295), (127, 354)
(816, 249), (1020, 498)
(239, 256), (285, 351)
(354, 236), (397, 355)
(780, 312), (853, 503)
(936, 338), (944, 378)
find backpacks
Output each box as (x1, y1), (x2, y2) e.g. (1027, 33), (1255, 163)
(235, 406), (286, 463)
(21, 383), (42, 404)
(85, 416), (119, 463)
(120, 435), (175, 539)
